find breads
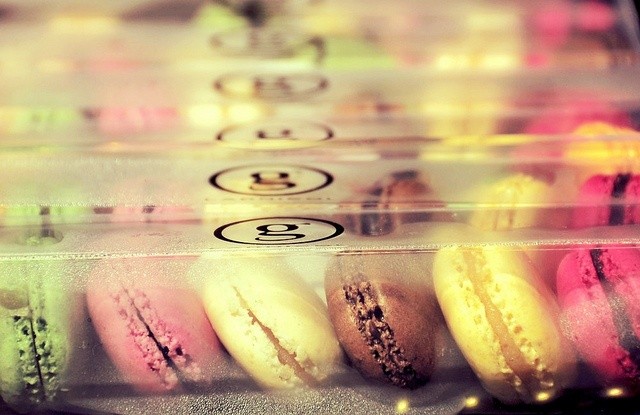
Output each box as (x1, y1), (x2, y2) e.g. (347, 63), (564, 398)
(85, 257), (222, 394)
(197, 250), (345, 392)
(555, 243), (640, 382)
(432, 227), (581, 405)
(323, 249), (446, 390)
(0, 257), (73, 413)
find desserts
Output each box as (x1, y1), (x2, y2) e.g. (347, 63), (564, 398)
(0, 1), (639, 245)
